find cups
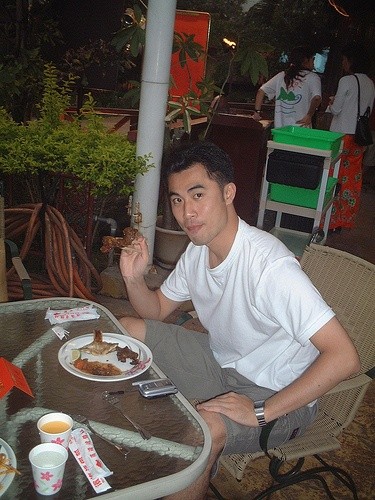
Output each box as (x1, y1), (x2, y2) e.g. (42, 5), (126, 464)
(37, 412), (74, 451)
(29, 442), (69, 496)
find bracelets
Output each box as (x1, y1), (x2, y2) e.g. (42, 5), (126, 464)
(253, 109), (261, 113)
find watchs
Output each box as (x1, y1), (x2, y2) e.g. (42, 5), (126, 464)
(254, 399), (267, 426)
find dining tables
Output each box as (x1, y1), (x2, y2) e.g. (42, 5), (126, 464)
(0, 296), (211, 500)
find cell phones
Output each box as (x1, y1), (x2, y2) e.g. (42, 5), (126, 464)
(131, 378), (178, 400)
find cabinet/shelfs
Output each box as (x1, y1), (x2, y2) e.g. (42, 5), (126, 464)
(258, 141), (342, 259)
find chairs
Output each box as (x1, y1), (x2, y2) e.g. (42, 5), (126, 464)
(0, 196), (34, 304)
(192, 245), (375, 500)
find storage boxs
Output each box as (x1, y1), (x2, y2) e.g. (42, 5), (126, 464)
(270, 125), (346, 159)
(266, 150), (325, 189)
(269, 177), (339, 210)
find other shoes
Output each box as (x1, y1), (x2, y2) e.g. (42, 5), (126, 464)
(328, 227), (341, 233)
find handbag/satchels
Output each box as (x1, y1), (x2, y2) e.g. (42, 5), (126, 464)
(356, 105), (373, 145)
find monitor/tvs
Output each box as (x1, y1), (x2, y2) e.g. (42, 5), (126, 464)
(279, 48), (330, 74)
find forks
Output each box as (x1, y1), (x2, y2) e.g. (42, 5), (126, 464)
(101, 392), (151, 440)
(70, 414), (131, 455)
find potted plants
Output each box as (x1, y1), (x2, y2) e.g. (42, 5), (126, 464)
(108, 0), (282, 270)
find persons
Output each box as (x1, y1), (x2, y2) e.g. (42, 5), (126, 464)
(252, 45), (322, 128)
(320, 43), (375, 234)
(117, 142), (363, 500)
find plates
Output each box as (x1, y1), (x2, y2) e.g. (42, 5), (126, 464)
(0, 437), (18, 497)
(58, 333), (153, 381)
